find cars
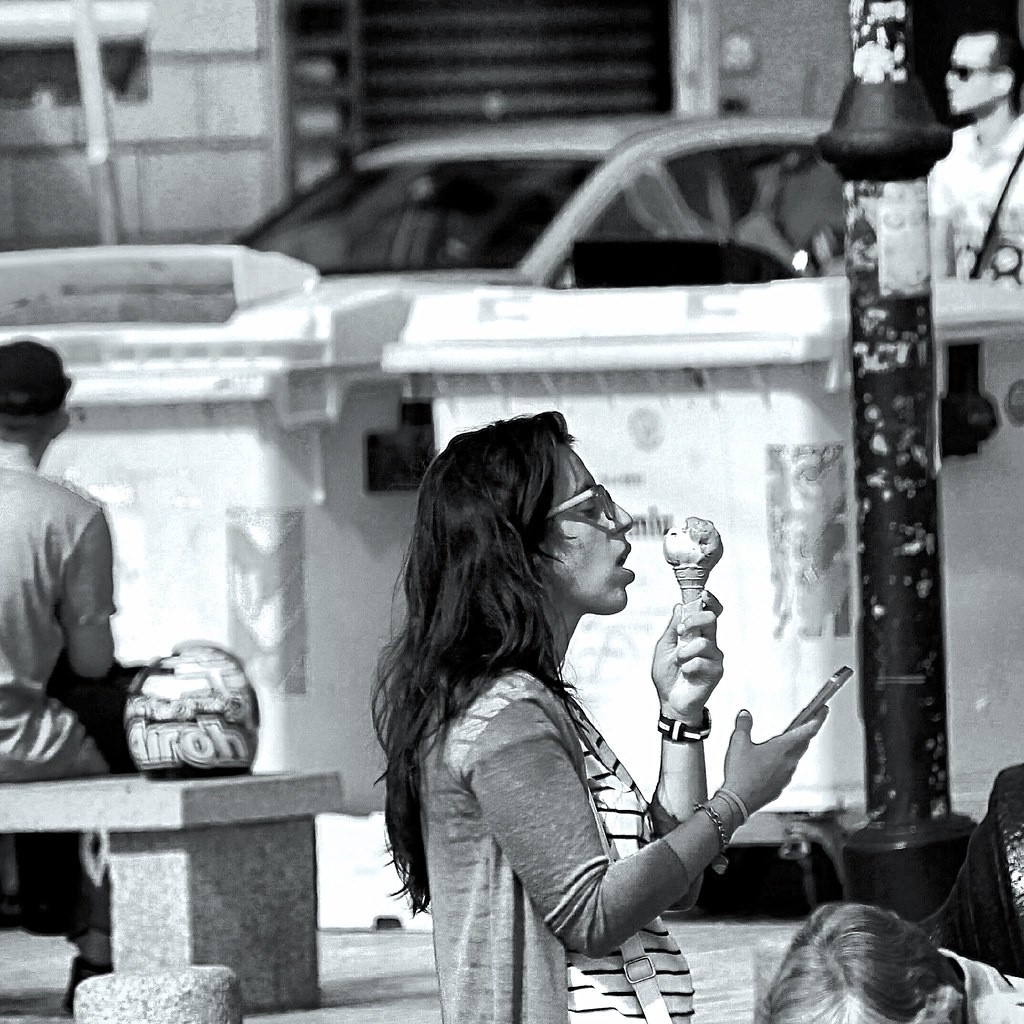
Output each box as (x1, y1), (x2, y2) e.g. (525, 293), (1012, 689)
(225, 112), (850, 290)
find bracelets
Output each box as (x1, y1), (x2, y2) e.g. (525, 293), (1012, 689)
(693, 802), (728, 875)
(714, 786), (748, 833)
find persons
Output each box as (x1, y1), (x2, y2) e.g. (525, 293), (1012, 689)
(371, 411), (829, 1023)
(928, 16), (1022, 277)
(0, 338), (130, 1013)
(758, 901), (1024, 1024)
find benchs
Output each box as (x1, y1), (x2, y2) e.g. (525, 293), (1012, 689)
(0, 772), (341, 1012)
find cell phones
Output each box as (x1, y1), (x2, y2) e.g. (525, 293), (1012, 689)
(784, 667), (854, 734)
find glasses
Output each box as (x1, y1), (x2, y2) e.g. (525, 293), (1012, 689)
(545, 484), (614, 520)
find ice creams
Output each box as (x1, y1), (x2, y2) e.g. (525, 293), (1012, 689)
(663, 516), (723, 604)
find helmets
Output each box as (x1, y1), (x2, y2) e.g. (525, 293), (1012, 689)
(123, 640), (259, 778)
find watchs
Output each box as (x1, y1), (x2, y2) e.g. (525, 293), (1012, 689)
(658, 707), (711, 743)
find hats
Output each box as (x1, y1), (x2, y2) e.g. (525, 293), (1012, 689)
(0, 341), (71, 415)
